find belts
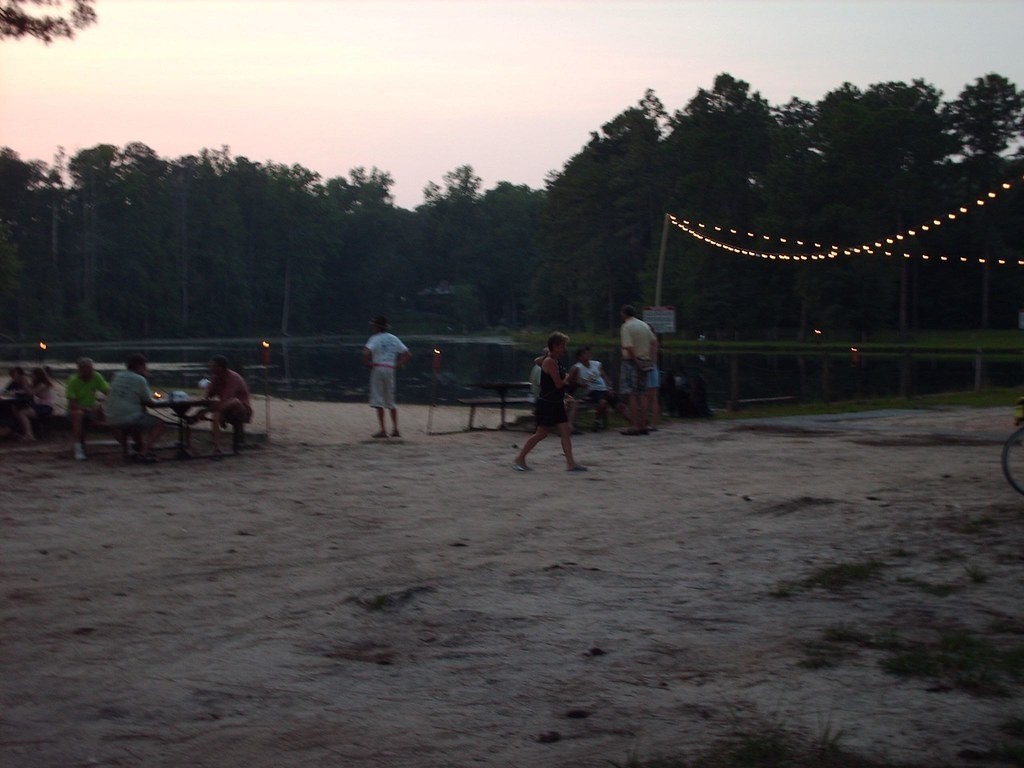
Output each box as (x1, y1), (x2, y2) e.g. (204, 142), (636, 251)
(367, 364), (397, 369)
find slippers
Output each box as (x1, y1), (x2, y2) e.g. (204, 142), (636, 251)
(565, 464), (588, 472)
(507, 462), (532, 471)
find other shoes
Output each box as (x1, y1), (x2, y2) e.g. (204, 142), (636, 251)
(144, 452), (160, 463)
(18, 432), (35, 441)
(592, 419), (600, 432)
(73, 443), (87, 461)
(390, 433), (400, 437)
(649, 425), (655, 431)
(212, 448), (224, 461)
(618, 428), (647, 435)
(372, 432), (388, 438)
(132, 453), (146, 465)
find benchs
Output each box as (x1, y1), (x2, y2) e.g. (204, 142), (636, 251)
(208, 409), (253, 450)
(456, 397), (609, 431)
(81, 420), (143, 466)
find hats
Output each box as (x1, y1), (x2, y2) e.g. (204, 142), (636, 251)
(368, 315), (392, 330)
(125, 353), (150, 363)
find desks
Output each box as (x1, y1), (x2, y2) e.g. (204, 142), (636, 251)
(464, 380), (532, 431)
(148, 395), (221, 460)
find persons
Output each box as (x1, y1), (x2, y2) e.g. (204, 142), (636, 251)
(530, 305), (659, 436)
(0, 353), (166, 463)
(511, 331), (590, 473)
(186, 355), (250, 460)
(362, 315), (412, 437)
